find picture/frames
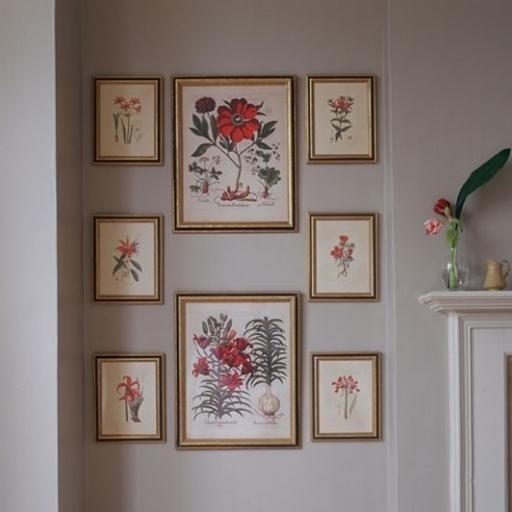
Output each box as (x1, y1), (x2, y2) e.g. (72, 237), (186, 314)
(306, 75), (378, 164)
(307, 210), (379, 303)
(173, 290), (302, 450)
(92, 352), (166, 445)
(312, 352), (384, 442)
(91, 214), (165, 306)
(91, 73), (165, 167)
(171, 74), (298, 233)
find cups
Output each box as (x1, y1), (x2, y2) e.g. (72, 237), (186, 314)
(481, 256), (510, 291)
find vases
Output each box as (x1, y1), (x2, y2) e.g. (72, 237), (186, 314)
(439, 249), (470, 290)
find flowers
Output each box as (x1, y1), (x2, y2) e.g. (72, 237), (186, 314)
(423, 147), (511, 287)
